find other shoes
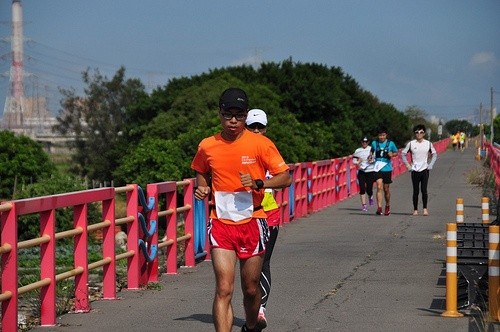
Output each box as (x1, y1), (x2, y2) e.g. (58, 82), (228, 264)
(413, 210), (418, 215)
(423, 210), (428, 215)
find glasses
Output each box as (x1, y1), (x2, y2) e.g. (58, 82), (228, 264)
(247, 123), (266, 129)
(223, 112), (246, 121)
(414, 131), (424, 134)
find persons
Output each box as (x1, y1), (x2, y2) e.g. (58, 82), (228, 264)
(450, 131), (465, 152)
(190, 89), (290, 332)
(367, 128), (398, 216)
(401, 125), (437, 216)
(353, 137), (375, 212)
(240, 109), (276, 328)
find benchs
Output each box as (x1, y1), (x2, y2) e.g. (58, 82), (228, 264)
(457, 222), (491, 317)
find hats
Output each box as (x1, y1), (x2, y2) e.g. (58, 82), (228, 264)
(219, 88), (249, 111)
(245, 109), (267, 125)
(361, 136), (369, 142)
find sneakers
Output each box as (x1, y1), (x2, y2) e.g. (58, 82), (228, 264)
(241, 322), (262, 332)
(385, 205), (390, 216)
(362, 205), (367, 212)
(369, 196), (373, 206)
(257, 307), (267, 329)
(376, 208), (383, 214)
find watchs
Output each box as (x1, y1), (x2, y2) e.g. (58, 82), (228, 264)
(254, 179), (264, 192)
